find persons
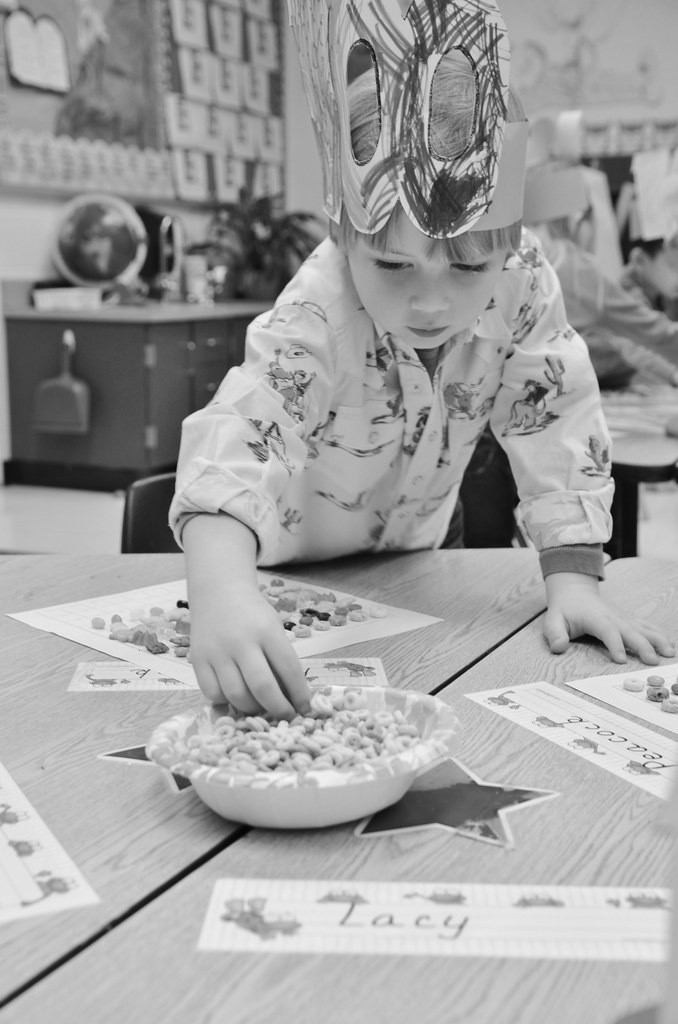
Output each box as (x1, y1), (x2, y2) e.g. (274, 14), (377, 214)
(491, 109), (678, 392)
(161, 52), (678, 718)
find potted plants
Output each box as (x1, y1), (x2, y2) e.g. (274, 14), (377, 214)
(192, 152), (323, 302)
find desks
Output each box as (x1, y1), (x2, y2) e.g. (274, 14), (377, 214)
(595, 382), (677, 560)
(0, 556), (678, 1024)
(0, 544), (609, 1024)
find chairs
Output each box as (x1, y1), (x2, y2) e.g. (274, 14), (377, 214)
(120, 472), (184, 554)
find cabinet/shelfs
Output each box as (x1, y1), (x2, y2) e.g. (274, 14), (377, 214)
(2, 304), (276, 471)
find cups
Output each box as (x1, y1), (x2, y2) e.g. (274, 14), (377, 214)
(184, 256), (208, 302)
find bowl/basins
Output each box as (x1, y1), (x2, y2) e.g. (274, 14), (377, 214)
(148, 686), (463, 829)
